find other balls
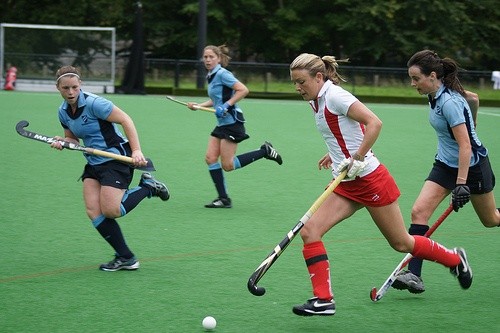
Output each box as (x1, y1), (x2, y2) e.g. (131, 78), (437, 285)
(202, 316), (217, 330)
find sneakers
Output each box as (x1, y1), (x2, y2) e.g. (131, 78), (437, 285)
(139, 172), (169, 201)
(99, 253), (139, 272)
(391, 269), (426, 294)
(261, 141), (283, 165)
(204, 197), (232, 208)
(292, 297), (336, 316)
(450, 247), (473, 289)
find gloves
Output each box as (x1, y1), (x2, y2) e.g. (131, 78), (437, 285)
(452, 184), (470, 212)
(215, 102), (233, 118)
(338, 154), (365, 178)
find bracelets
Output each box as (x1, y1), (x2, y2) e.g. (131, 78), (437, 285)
(457, 177), (467, 182)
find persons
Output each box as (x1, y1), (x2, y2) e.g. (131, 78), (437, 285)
(188, 45), (283, 208)
(51, 66), (169, 273)
(289, 52), (472, 315)
(391, 50), (500, 294)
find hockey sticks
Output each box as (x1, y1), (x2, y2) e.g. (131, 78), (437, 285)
(166, 95), (215, 112)
(246, 167), (350, 296)
(16, 119), (149, 167)
(369, 202), (455, 301)
